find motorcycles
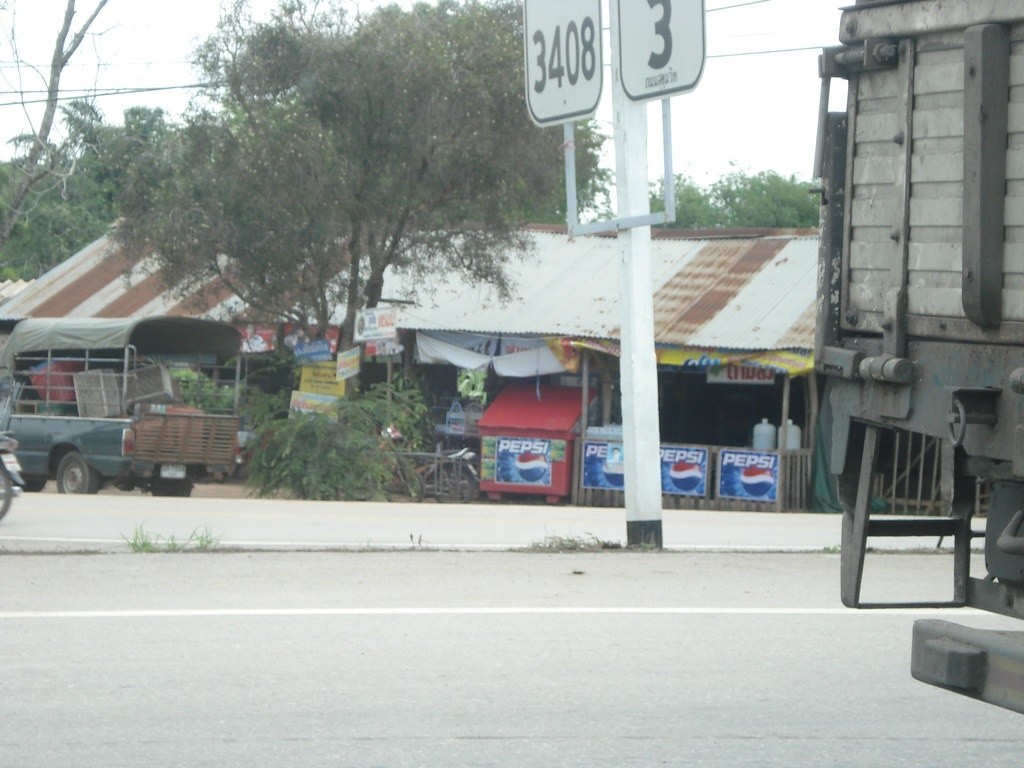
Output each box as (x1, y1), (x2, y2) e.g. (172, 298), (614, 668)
(0, 430), (26, 521)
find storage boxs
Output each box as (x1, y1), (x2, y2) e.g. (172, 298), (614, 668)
(73, 367), (121, 418)
(118, 361), (174, 404)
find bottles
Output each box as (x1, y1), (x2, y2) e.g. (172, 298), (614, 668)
(752, 417), (777, 450)
(586, 421), (623, 441)
(777, 419), (801, 451)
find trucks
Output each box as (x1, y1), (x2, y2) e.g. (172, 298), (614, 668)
(810, 0), (1024, 715)
(0, 315), (243, 497)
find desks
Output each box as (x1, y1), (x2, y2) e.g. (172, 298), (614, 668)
(370, 448), (444, 497)
